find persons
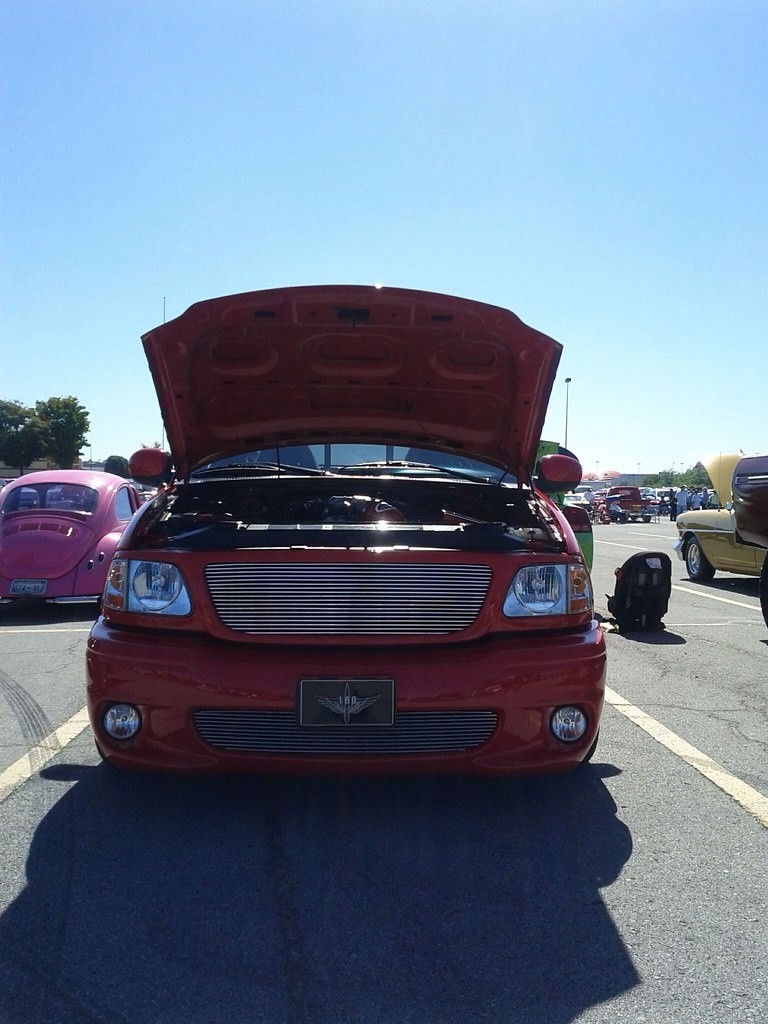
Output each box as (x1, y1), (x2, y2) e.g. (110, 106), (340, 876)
(659, 497), (668, 509)
(669, 489), (677, 521)
(709, 490), (720, 508)
(688, 486), (696, 495)
(585, 489), (594, 504)
(701, 487), (709, 509)
(675, 486), (690, 515)
(610, 500), (623, 524)
(691, 490), (701, 510)
(105, 455), (131, 479)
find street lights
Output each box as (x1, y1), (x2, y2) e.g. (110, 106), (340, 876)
(565, 378), (571, 448)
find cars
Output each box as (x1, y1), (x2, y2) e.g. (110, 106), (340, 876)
(564, 485), (718, 523)
(0, 470), (143, 607)
(674, 453), (768, 581)
(532, 440), (593, 574)
(87, 285), (607, 776)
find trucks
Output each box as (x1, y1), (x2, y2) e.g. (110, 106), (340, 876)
(605, 485), (659, 523)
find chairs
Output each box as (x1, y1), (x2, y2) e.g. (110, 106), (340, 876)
(593, 504), (667, 524)
